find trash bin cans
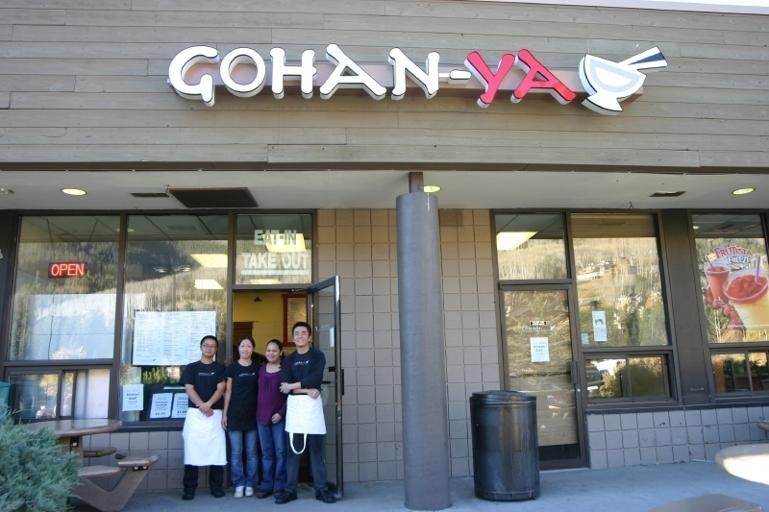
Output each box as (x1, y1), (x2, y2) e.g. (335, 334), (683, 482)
(469, 389), (540, 502)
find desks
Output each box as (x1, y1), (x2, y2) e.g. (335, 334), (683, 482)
(12, 417), (124, 512)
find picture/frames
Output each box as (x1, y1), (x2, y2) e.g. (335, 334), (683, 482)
(281, 293), (307, 348)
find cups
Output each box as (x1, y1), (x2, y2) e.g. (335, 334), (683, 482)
(705, 266), (730, 304)
(721, 267), (769, 333)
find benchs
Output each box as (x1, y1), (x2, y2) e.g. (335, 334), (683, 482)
(76, 463), (121, 482)
(80, 445), (117, 457)
(115, 448), (161, 512)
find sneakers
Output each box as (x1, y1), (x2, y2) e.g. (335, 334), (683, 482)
(234, 485), (244, 498)
(245, 487), (254, 497)
(316, 490), (335, 503)
(256, 488), (269, 498)
(182, 491), (194, 500)
(273, 490), (296, 504)
(213, 489), (225, 497)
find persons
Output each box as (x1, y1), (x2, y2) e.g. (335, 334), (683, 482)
(221, 336), (261, 499)
(256, 339), (285, 498)
(275, 321), (337, 504)
(179, 335), (225, 500)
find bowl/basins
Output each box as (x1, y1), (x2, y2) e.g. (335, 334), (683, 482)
(566, 56), (653, 119)
